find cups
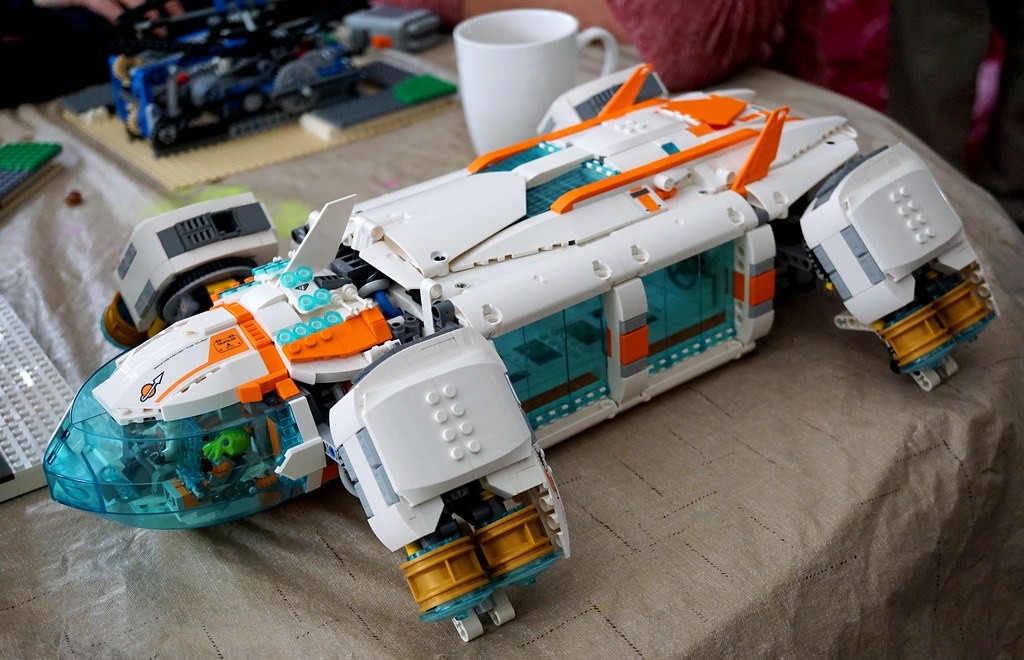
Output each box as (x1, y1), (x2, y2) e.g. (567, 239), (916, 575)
(452, 8), (620, 160)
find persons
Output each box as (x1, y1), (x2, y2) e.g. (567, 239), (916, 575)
(132, 420), (181, 494)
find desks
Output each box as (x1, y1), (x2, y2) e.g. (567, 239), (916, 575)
(0, 12), (1023, 660)
